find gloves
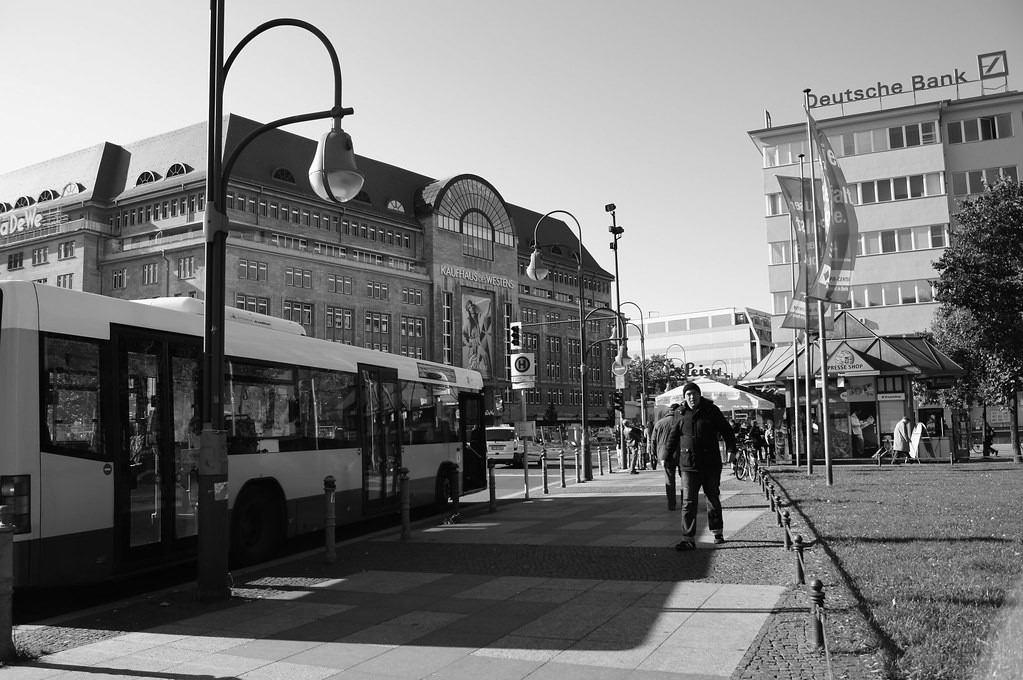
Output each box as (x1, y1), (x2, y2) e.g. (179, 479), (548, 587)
(662, 460), (672, 477)
(727, 451), (737, 470)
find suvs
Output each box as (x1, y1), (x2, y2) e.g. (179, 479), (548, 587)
(484, 424), (546, 469)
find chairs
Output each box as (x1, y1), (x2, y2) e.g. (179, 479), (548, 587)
(91, 413), (450, 453)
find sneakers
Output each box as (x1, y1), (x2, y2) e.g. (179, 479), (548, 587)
(713, 534), (724, 544)
(675, 540), (696, 550)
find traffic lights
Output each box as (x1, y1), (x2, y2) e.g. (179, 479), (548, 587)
(613, 391), (624, 412)
(510, 322), (523, 350)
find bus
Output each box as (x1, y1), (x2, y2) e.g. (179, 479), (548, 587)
(0, 279), (505, 613)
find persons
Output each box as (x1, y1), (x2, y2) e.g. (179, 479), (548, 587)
(616, 419), (658, 475)
(985, 421), (999, 456)
(728, 417), (776, 464)
(890, 416), (916, 465)
(462, 300), (492, 379)
(851, 407), (866, 457)
(927, 413), (937, 437)
(661, 383), (739, 550)
(651, 402), (684, 510)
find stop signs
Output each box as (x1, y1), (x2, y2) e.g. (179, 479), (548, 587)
(612, 360), (628, 377)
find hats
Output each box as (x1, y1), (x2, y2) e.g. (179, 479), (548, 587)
(683, 383), (701, 398)
(670, 404), (680, 411)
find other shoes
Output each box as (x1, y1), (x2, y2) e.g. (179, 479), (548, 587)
(631, 470), (639, 474)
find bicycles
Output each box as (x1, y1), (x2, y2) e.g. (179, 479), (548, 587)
(735, 439), (758, 482)
(969, 435), (984, 454)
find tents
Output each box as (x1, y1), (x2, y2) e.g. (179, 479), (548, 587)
(654, 377), (775, 418)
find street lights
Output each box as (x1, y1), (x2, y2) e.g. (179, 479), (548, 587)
(662, 342), (688, 387)
(609, 301), (672, 428)
(526, 209), (632, 482)
(192, 0), (366, 609)
